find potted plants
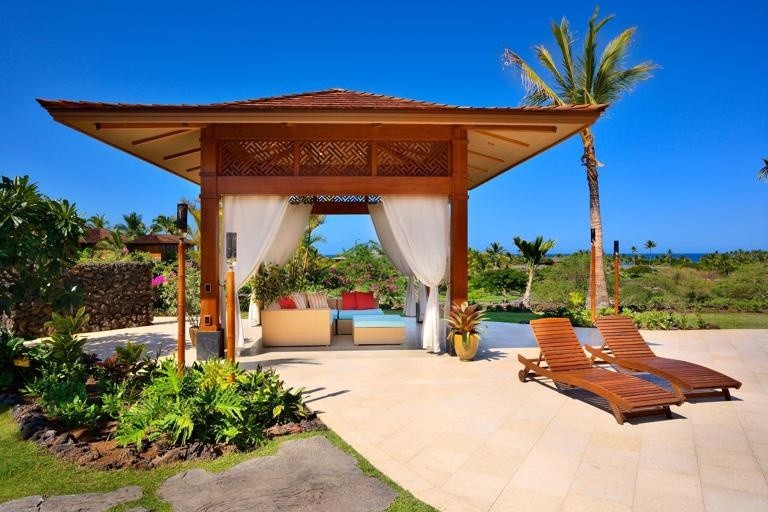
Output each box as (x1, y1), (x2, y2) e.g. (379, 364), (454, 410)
(440, 302), (492, 362)
(159, 262), (202, 347)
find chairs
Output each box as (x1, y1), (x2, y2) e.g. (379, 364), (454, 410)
(584, 316), (742, 405)
(517, 316), (682, 425)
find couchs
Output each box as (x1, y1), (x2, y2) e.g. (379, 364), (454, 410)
(261, 298), (338, 348)
(337, 298), (383, 334)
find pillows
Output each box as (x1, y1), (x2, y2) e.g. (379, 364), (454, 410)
(306, 291), (332, 311)
(278, 297), (296, 309)
(266, 302), (281, 311)
(341, 291), (357, 310)
(356, 290), (378, 310)
(291, 291), (309, 310)
